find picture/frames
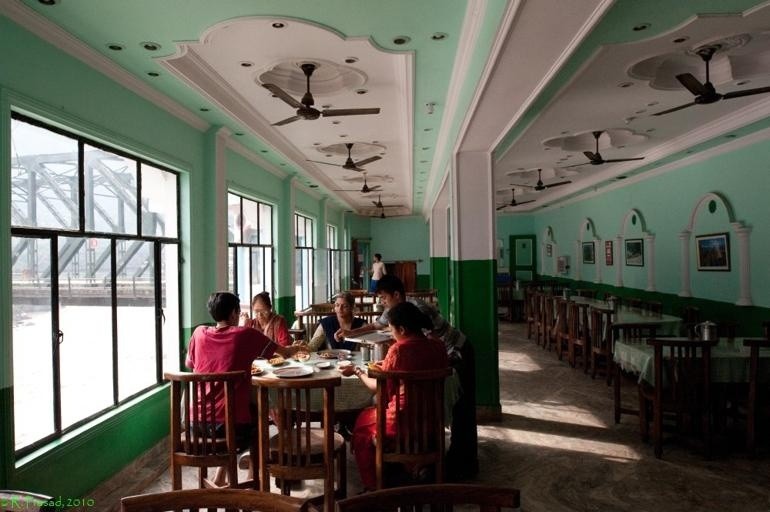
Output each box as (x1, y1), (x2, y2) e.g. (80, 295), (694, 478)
(546, 232), (731, 274)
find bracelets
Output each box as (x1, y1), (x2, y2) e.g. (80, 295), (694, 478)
(353, 368), (365, 377)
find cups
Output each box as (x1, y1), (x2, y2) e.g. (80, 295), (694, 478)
(359, 342), (382, 363)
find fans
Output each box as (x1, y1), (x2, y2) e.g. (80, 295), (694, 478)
(496, 188), (535, 210)
(560, 131), (645, 168)
(509, 169), (572, 190)
(261, 64), (404, 218)
(652, 43), (770, 117)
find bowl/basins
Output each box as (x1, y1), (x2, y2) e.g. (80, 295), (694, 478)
(266, 358), (285, 364)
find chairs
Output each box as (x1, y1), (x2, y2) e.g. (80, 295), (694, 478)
(0, 488), (56, 511)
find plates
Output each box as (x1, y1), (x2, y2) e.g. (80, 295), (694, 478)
(315, 361), (332, 368)
(292, 352), (311, 361)
(316, 349), (352, 357)
(336, 360), (352, 367)
(251, 363), (264, 376)
(270, 364), (314, 378)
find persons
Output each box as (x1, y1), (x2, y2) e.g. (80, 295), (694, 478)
(334, 274), (479, 475)
(300, 290), (369, 352)
(241, 290), (293, 348)
(336, 301), (454, 494)
(370, 253), (388, 290)
(185, 292), (307, 491)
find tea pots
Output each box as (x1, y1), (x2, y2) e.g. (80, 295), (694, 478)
(694, 320), (718, 346)
(608, 296), (618, 314)
(563, 288), (571, 300)
